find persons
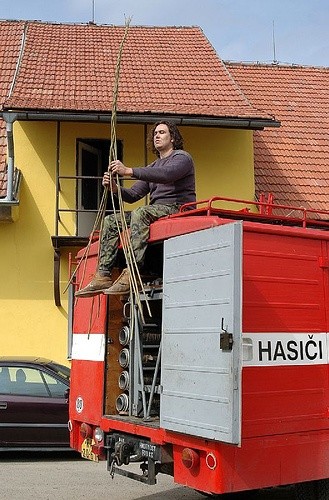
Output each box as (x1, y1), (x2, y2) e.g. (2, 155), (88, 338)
(74, 120), (196, 298)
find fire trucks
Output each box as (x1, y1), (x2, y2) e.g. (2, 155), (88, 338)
(61, 191), (329, 496)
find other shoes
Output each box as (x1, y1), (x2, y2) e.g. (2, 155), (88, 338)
(103, 268), (143, 296)
(73, 277), (114, 298)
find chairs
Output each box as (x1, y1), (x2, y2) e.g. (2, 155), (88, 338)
(16, 369), (26, 385)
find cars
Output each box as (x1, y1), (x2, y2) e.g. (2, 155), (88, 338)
(0, 357), (142, 464)
